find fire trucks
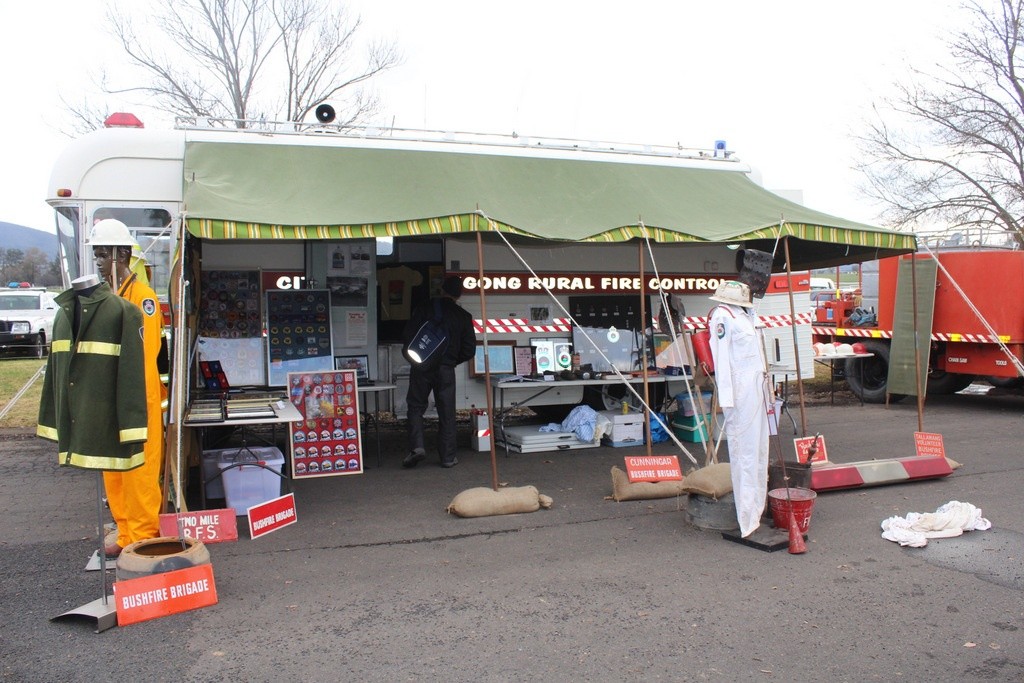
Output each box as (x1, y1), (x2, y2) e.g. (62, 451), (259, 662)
(812, 249), (1024, 404)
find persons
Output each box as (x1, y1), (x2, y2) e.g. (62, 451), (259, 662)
(84, 219), (164, 558)
(708, 279), (771, 539)
(402, 276), (479, 467)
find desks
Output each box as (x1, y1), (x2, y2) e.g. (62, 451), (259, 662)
(182, 396), (304, 511)
(474, 375), (666, 458)
(243, 380), (396, 467)
(815, 352), (877, 407)
(660, 369), (807, 444)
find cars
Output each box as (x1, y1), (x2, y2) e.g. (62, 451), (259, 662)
(0, 281), (60, 358)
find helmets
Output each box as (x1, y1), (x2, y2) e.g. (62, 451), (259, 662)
(706, 280), (756, 308)
(83, 217), (138, 246)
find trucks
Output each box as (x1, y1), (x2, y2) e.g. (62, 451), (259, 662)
(44, 112), (750, 451)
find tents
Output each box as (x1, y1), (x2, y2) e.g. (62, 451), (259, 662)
(177, 131), (916, 512)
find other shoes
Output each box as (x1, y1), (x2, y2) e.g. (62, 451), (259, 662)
(95, 542), (125, 559)
(443, 455), (459, 467)
(401, 446), (428, 467)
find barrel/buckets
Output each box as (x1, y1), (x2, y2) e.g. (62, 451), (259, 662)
(767, 487), (817, 534)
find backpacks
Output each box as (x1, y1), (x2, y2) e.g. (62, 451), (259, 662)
(401, 314), (454, 369)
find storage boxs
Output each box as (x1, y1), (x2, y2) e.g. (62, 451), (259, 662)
(597, 408), (646, 448)
(769, 396), (782, 436)
(671, 393), (728, 443)
(202, 447), (285, 517)
(470, 414), (491, 452)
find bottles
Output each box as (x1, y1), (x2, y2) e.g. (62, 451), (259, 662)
(333, 246), (344, 268)
(622, 401), (628, 414)
(471, 404), (477, 416)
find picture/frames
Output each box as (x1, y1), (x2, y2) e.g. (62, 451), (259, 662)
(333, 355), (369, 380)
(570, 295), (658, 374)
(528, 336), (574, 377)
(653, 331), (699, 372)
(469, 340), (517, 378)
